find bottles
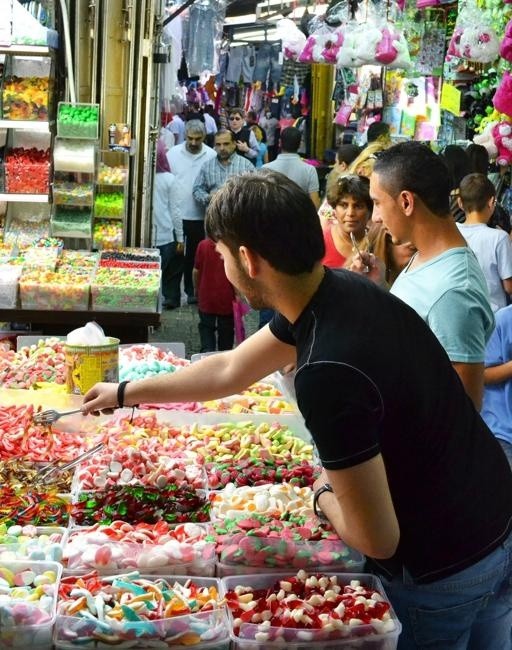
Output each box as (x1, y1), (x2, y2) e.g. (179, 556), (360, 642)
(123, 125), (130, 147)
(109, 123), (117, 143)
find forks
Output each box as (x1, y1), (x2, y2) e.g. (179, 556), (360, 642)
(33, 405), (99, 426)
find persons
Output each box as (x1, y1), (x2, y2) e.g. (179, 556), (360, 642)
(153, 75), (319, 350)
(314, 121), (512, 465)
(82, 168), (512, 650)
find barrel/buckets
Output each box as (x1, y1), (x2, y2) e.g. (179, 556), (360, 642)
(64, 335), (122, 397)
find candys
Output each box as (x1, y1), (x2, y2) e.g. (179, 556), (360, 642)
(0, 34), (162, 310)
(0, 334), (397, 650)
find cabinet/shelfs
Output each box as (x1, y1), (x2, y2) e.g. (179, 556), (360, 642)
(0, 44), (60, 204)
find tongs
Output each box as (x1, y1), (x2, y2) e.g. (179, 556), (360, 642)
(349, 227), (374, 272)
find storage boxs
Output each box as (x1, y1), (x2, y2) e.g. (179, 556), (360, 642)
(16, 264), (90, 311)
(1, 334), (403, 649)
(92, 267), (162, 312)
(0, 265), (23, 310)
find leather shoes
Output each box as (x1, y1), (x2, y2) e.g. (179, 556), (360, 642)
(186, 296), (197, 303)
(161, 298), (181, 308)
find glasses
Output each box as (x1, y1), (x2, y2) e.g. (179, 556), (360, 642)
(229, 117), (240, 120)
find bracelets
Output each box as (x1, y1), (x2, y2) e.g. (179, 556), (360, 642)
(117, 381), (139, 409)
(313, 483), (334, 518)
